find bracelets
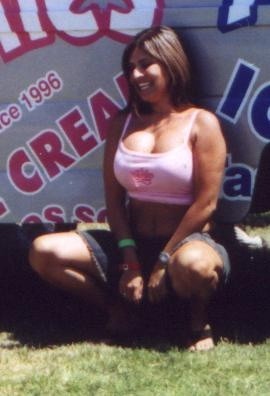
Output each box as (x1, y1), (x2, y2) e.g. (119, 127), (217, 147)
(116, 262), (143, 272)
(118, 239), (138, 250)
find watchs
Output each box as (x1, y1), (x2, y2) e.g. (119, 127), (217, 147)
(159, 252), (171, 266)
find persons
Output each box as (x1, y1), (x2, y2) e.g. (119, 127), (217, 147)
(28, 23), (232, 353)
